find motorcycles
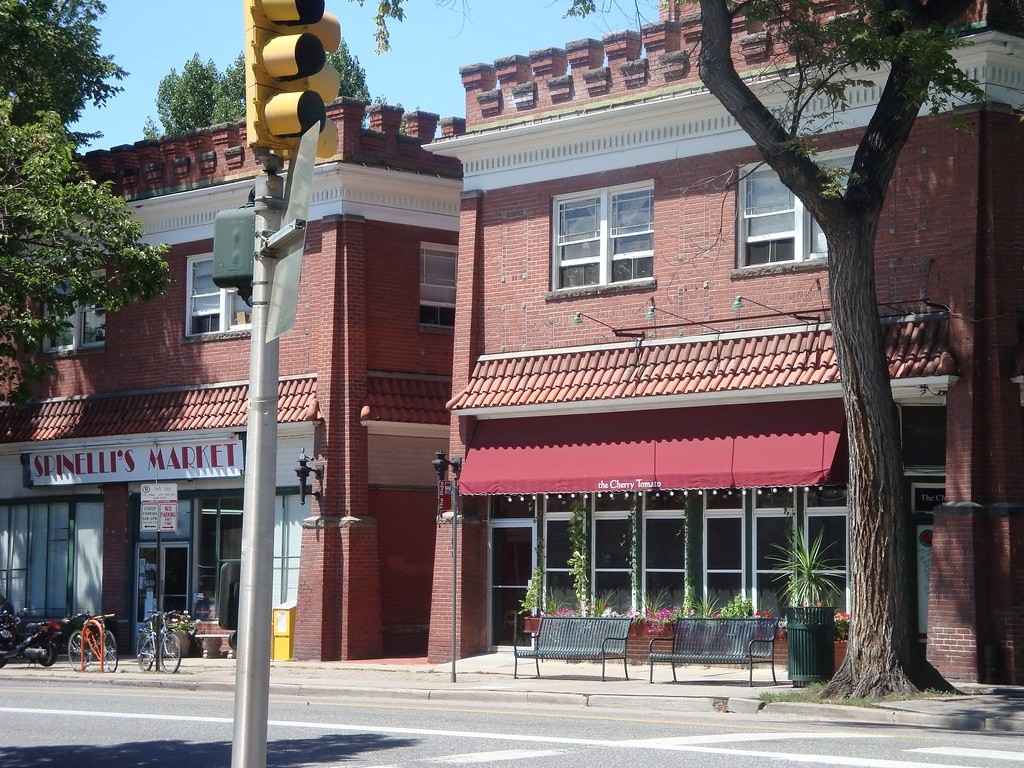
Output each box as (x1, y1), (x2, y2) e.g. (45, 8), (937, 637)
(0, 600), (61, 668)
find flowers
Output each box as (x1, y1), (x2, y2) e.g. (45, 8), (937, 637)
(165, 610), (202, 631)
(540, 603), (849, 626)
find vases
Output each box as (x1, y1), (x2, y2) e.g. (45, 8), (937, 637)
(524, 616), (787, 641)
(838, 629), (846, 638)
(170, 631), (191, 658)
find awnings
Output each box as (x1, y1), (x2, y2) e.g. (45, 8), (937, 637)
(459, 398), (855, 494)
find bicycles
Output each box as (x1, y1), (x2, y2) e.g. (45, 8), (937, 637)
(67, 613), (118, 672)
(136, 610), (182, 674)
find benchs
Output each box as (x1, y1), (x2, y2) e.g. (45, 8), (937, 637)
(513, 616), (633, 682)
(649, 617), (780, 687)
(195, 634), (231, 658)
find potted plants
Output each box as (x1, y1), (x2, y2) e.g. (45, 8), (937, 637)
(763, 526), (849, 688)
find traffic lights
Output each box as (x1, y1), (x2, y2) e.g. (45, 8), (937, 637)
(245, 1), (340, 158)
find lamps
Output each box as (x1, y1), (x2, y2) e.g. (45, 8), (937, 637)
(570, 311), (617, 332)
(731, 295), (810, 324)
(644, 306), (721, 334)
